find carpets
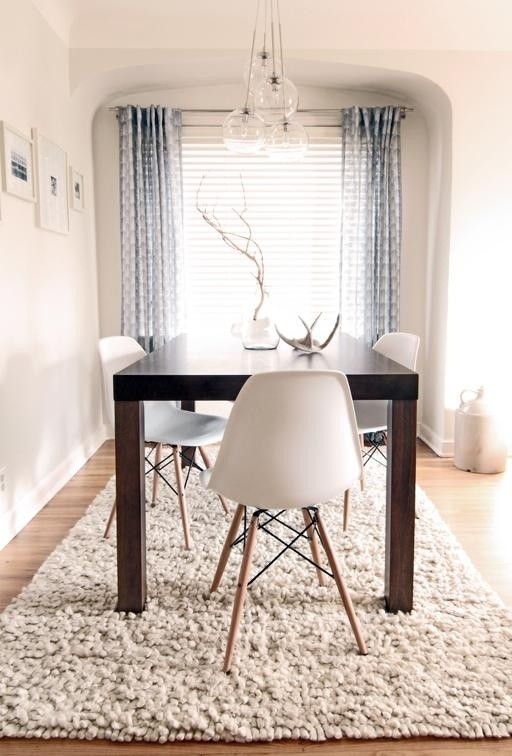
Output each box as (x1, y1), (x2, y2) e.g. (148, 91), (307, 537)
(1, 446), (512, 744)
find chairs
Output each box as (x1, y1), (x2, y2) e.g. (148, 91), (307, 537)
(96, 336), (229, 549)
(343, 332), (420, 532)
(210, 369), (368, 671)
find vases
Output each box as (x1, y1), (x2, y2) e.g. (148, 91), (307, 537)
(240, 313), (280, 349)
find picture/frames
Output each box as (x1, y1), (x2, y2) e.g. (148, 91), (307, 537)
(32, 127), (72, 235)
(69, 165), (85, 212)
(1, 120), (36, 203)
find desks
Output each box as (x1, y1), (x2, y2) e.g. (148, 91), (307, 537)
(112, 331), (419, 612)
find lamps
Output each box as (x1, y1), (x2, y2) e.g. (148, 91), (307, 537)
(222, 0), (309, 160)
